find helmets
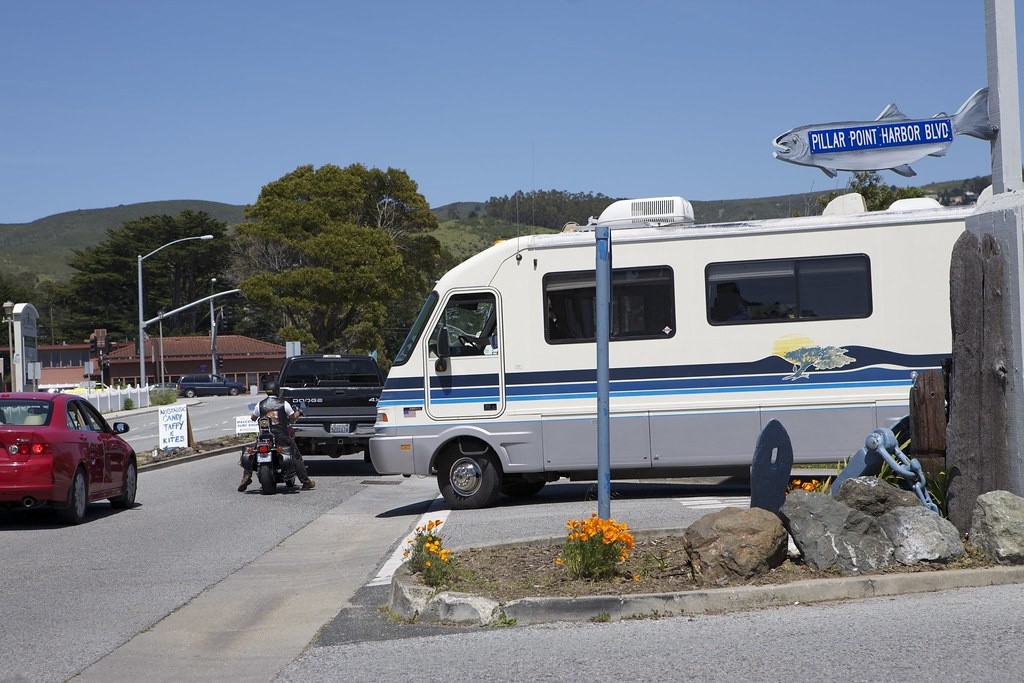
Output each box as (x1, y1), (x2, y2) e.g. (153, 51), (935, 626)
(265, 382), (279, 396)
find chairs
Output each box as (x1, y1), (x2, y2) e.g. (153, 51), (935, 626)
(23, 415), (45, 425)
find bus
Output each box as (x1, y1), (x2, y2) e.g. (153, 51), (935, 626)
(369, 197), (976, 509)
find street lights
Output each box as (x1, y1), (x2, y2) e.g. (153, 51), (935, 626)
(2, 302), (15, 392)
(210, 278), (217, 381)
(158, 312), (165, 389)
(138, 235), (213, 388)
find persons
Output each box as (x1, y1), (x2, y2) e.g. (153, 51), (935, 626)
(238, 382), (315, 490)
(464, 334), (497, 349)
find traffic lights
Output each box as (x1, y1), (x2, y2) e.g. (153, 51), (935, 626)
(105, 335), (112, 354)
(90, 338), (97, 354)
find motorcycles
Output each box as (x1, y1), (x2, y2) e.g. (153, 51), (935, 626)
(238, 404), (306, 495)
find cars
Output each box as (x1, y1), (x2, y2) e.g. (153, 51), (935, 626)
(70, 383), (109, 393)
(176, 374), (247, 398)
(0, 392), (137, 525)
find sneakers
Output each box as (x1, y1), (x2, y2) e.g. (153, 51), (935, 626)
(302, 478), (315, 490)
(238, 474), (252, 492)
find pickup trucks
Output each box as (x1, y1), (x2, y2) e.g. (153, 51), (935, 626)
(260, 353), (385, 463)
